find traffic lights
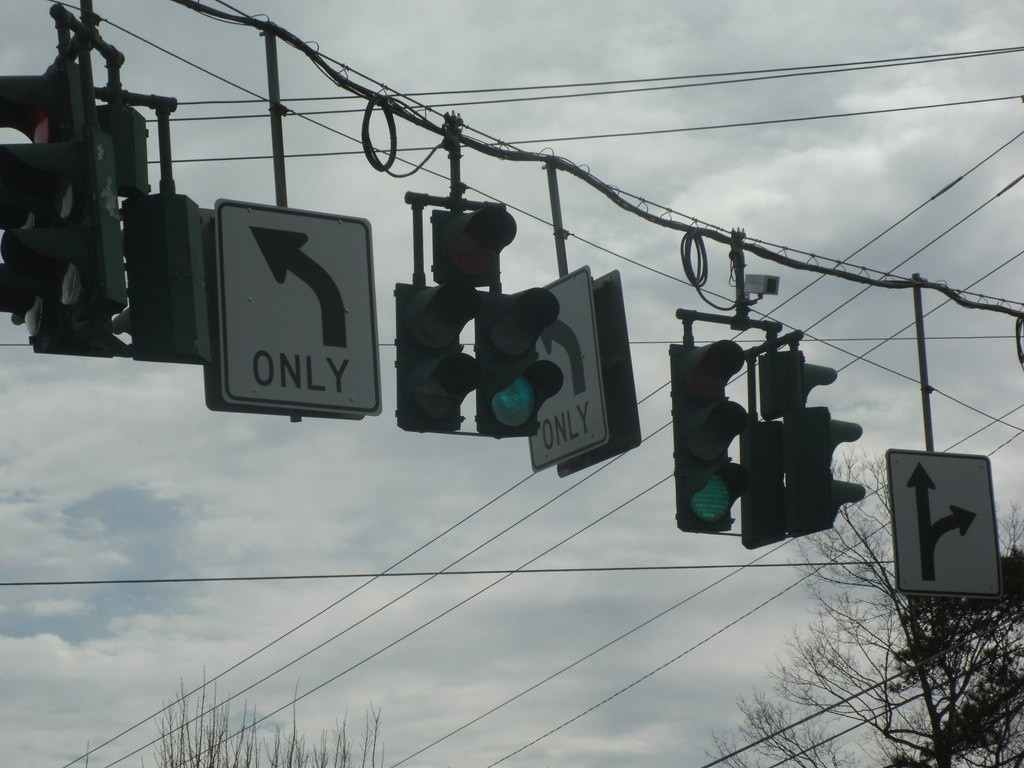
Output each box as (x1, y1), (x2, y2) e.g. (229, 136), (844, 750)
(476, 287), (562, 439)
(0, 53), (122, 345)
(397, 281), (485, 436)
(761, 348), (839, 422)
(782, 407), (866, 537)
(427, 204), (518, 290)
(668, 338), (748, 535)
